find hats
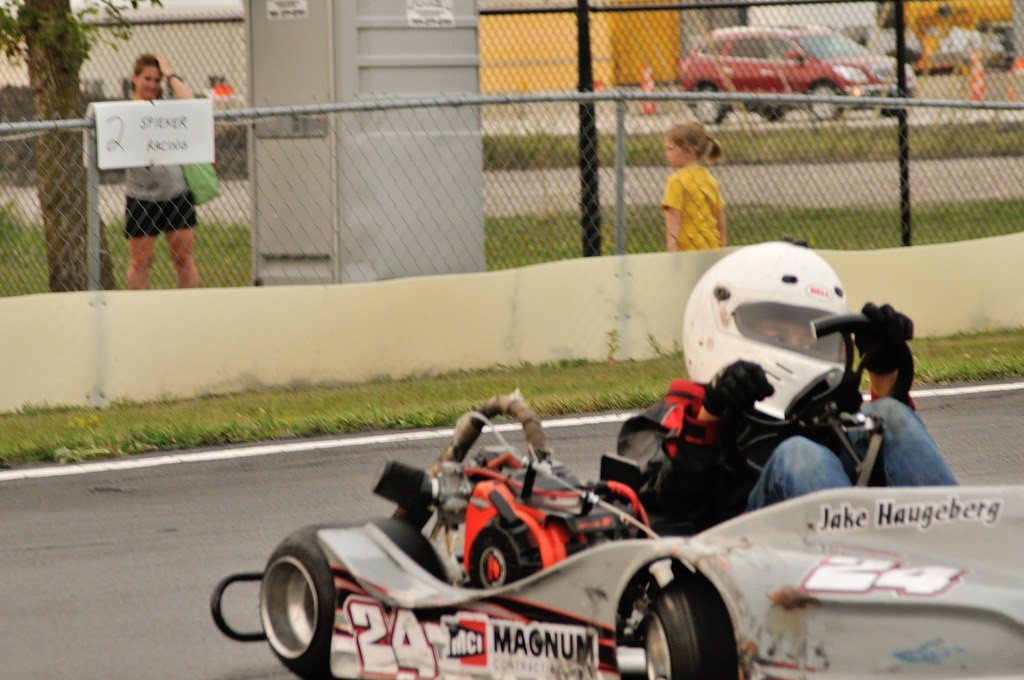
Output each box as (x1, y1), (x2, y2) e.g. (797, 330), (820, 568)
(680, 240), (847, 421)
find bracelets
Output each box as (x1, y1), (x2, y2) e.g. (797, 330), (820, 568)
(167, 73), (183, 97)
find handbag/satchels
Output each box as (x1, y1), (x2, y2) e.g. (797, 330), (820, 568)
(183, 162), (222, 204)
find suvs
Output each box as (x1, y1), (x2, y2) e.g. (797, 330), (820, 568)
(678, 21), (915, 118)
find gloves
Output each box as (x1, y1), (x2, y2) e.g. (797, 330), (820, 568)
(854, 302), (914, 376)
(701, 356), (774, 419)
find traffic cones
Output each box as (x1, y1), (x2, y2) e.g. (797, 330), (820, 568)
(637, 67), (662, 116)
(968, 43), (988, 102)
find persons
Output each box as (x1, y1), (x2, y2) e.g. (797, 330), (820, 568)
(660, 124), (728, 251)
(122, 52), (200, 289)
(613, 232), (959, 536)
